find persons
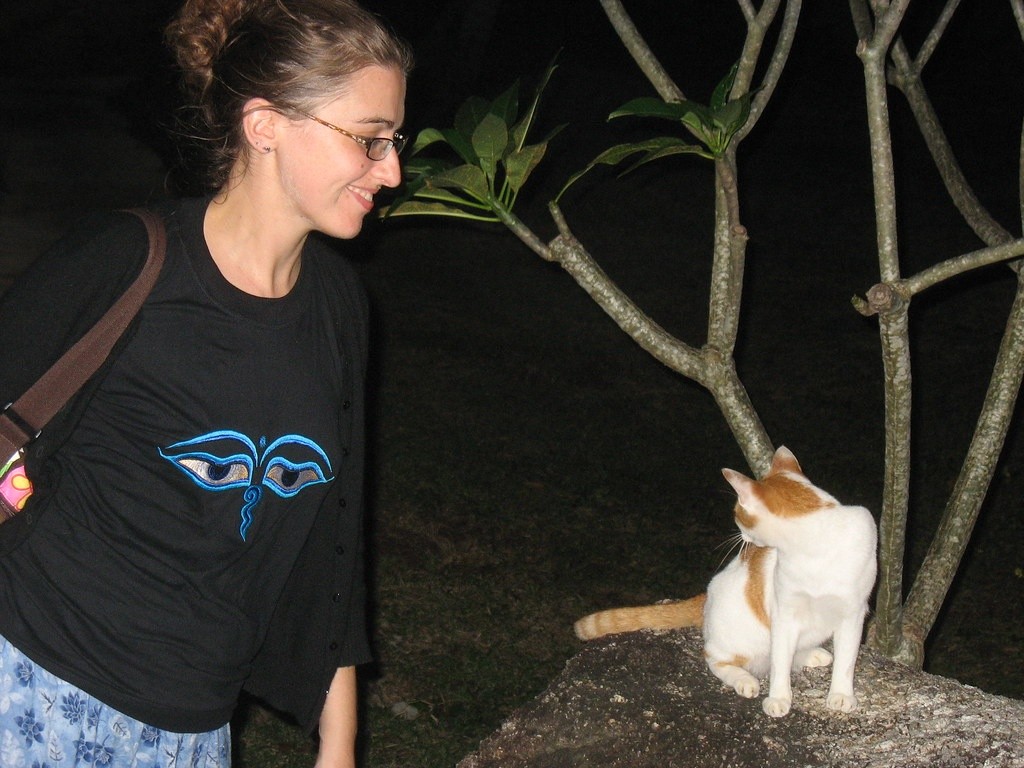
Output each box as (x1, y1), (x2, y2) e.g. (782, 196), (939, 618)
(0, 0), (406, 768)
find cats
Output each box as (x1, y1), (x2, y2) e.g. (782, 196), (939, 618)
(573, 444), (878, 718)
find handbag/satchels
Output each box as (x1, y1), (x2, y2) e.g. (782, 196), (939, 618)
(0, 420), (35, 524)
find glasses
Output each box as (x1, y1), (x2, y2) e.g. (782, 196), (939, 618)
(278, 97), (409, 160)
